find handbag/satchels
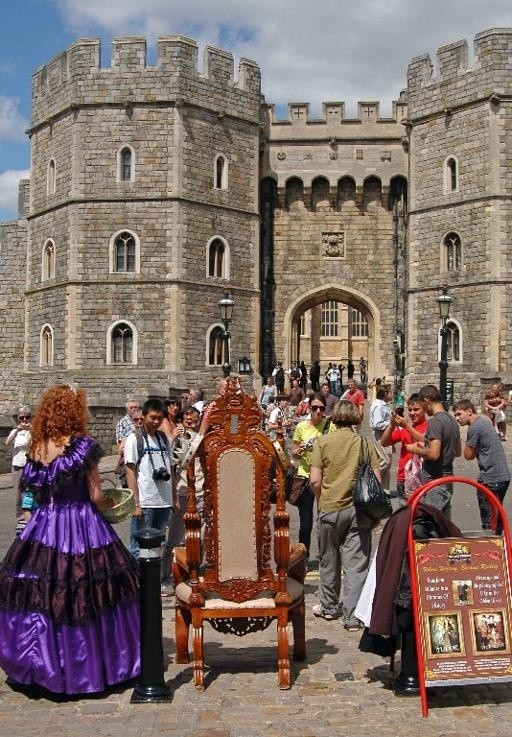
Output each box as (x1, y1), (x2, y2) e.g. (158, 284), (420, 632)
(352, 437), (394, 520)
(288, 475), (311, 506)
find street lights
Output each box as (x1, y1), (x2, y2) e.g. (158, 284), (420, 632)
(216, 291), (238, 381)
(432, 282), (458, 404)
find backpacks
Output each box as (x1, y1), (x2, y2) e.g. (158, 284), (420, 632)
(115, 430), (168, 489)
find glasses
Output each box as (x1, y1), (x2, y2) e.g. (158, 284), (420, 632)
(310, 406), (326, 412)
(19, 416), (31, 422)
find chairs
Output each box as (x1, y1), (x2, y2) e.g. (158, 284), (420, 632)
(166, 375), (309, 693)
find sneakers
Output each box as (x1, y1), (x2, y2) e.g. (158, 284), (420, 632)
(312, 605), (339, 620)
(162, 584), (175, 597)
(340, 616), (359, 632)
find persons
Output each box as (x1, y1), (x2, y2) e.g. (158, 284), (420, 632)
(441, 618), (454, 652)
(477, 615), (499, 650)
(0, 383), (139, 697)
(404, 385), (461, 520)
(456, 579), (470, 604)
(258, 357), (408, 503)
(381, 394), (429, 498)
(291, 394), (337, 574)
(482, 381), (512, 441)
(113, 377), (227, 456)
(123, 399), (181, 565)
(310, 401), (381, 629)
(452, 399), (511, 535)
(161, 405), (205, 598)
(5, 405), (34, 521)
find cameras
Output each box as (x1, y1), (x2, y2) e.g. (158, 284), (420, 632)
(21, 424), (30, 430)
(302, 439), (313, 450)
(152, 467), (170, 481)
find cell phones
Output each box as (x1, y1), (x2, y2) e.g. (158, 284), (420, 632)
(395, 407), (404, 418)
